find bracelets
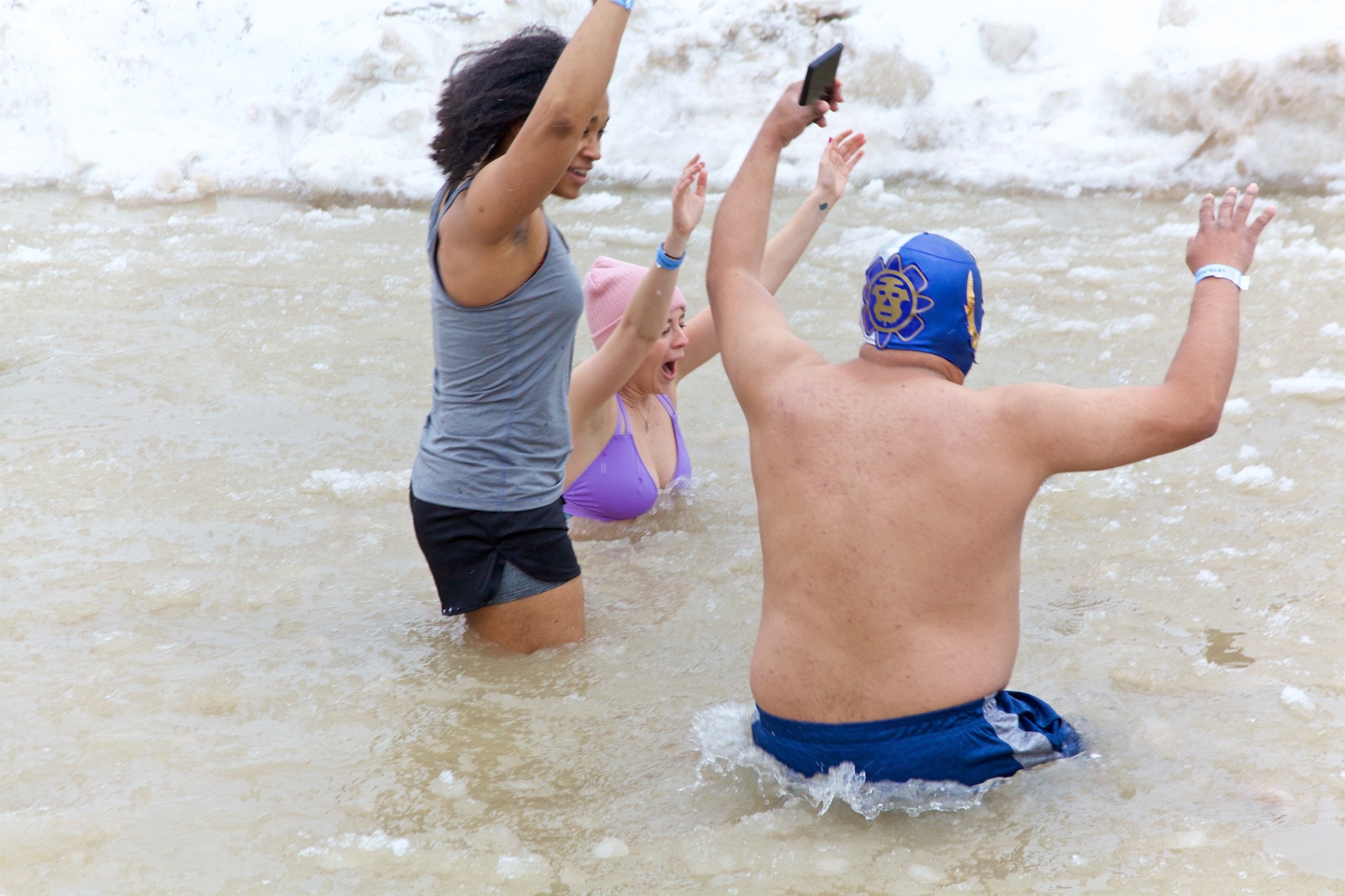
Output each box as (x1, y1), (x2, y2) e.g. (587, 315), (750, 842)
(663, 251), (680, 260)
(1195, 264), (1250, 290)
(614, 0), (635, 12)
(706, 80), (1275, 786)
(656, 242), (686, 269)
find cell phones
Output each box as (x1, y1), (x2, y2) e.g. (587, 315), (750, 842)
(799, 43), (841, 124)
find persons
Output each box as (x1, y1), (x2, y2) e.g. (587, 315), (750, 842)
(410, 0), (637, 655)
(562, 130), (867, 522)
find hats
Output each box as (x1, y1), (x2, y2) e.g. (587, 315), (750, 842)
(856, 232), (981, 376)
(584, 256), (685, 350)
